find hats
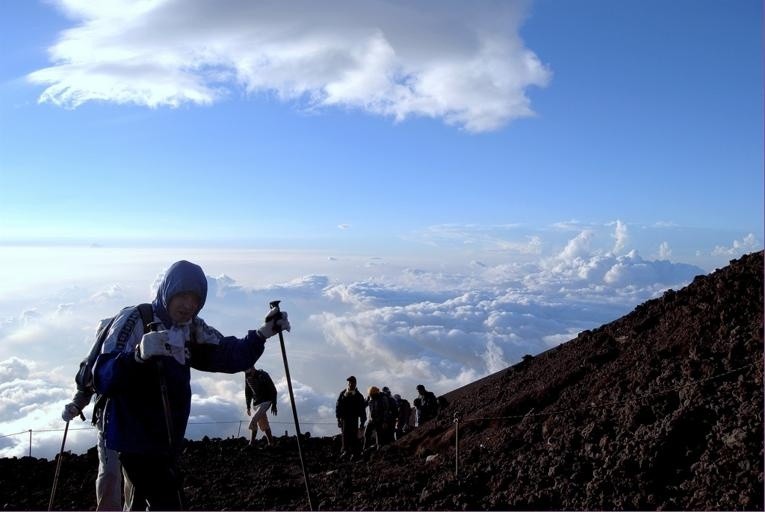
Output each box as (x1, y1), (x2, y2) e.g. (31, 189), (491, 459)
(345, 375), (356, 382)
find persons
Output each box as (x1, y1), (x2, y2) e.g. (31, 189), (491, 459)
(362, 384), (450, 450)
(335, 376), (367, 464)
(61, 316), (134, 512)
(91, 260), (290, 511)
(240, 365), (278, 451)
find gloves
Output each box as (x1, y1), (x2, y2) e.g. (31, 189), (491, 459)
(259, 308), (291, 339)
(60, 401), (80, 421)
(139, 329), (173, 360)
